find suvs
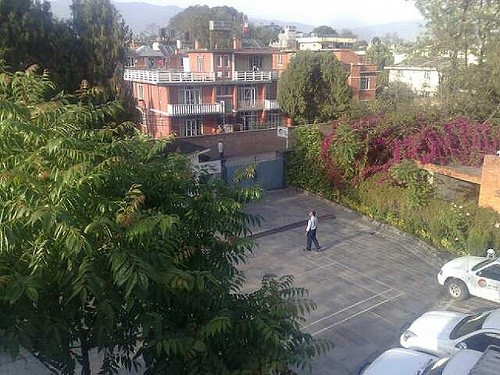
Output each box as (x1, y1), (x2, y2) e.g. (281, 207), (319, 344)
(358, 347), (500, 375)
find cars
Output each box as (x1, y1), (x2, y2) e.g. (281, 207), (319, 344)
(436, 253), (500, 305)
(400, 309), (500, 358)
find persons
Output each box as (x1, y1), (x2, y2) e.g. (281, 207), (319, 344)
(305, 211), (322, 252)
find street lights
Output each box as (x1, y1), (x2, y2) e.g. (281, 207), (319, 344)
(218, 139), (225, 181)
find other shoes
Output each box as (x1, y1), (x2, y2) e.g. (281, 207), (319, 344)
(316, 246), (321, 252)
(303, 247), (311, 251)
